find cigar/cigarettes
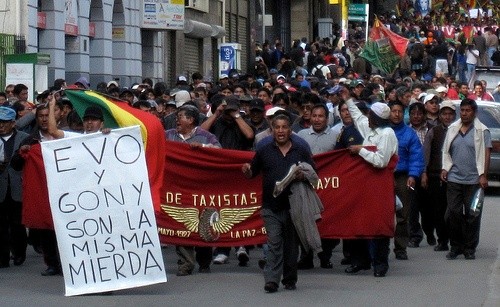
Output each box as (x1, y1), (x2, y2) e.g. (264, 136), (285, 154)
(408, 185), (414, 190)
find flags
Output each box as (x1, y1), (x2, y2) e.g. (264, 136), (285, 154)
(359, 19), (409, 77)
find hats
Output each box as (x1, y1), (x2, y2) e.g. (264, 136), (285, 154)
(247, 99), (266, 112)
(370, 102), (391, 120)
(356, 80), (366, 86)
(220, 73), (229, 80)
(270, 69), (279, 75)
(225, 94), (240, 112)
(107, 80), (119, 88)
(440, 100), (456, 111)
(119, 88), (135, 100)
(417, 89), (440, 105)
(239, 93), (253, 103)
(81, 106), (104, 122)
(75, 77), (90, 90)
(133, 85), (145, 92)
(435, 86), (448, 94)
(276, 75), (287, 81)
(264, 107), (286, 117)
(165, 76), (206, 109)
(0, 106), (16, 122)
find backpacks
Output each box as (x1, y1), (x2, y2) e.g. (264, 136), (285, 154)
(312, 65), (327, 81)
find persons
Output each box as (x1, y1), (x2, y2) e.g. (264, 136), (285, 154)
(0, 68), (500, 267)
(296, 103), (340, 270)
(241, 116), (317, 290)
(21, 107), (63, 277)
(441, 98), (493, 260)
(256, 2), (500, 84)
(421, 100), (458, 252)
(160, 104), (223, 276)
(49, 94), (112, 135)
(386, 100), (424, 261)
(337, 86), (398, 278)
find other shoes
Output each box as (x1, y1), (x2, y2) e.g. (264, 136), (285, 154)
(176, 239), (478, 293)
(0, 247), (64, 277)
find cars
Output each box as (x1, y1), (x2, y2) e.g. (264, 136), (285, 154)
(441, 99), (500, 181)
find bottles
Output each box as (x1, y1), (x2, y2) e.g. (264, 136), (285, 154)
(469, 187), (485, 217)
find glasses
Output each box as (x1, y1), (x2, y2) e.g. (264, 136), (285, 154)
(428, 101), (439, 105)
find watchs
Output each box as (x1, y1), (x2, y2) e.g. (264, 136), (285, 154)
(233, 113), (240, 119)
